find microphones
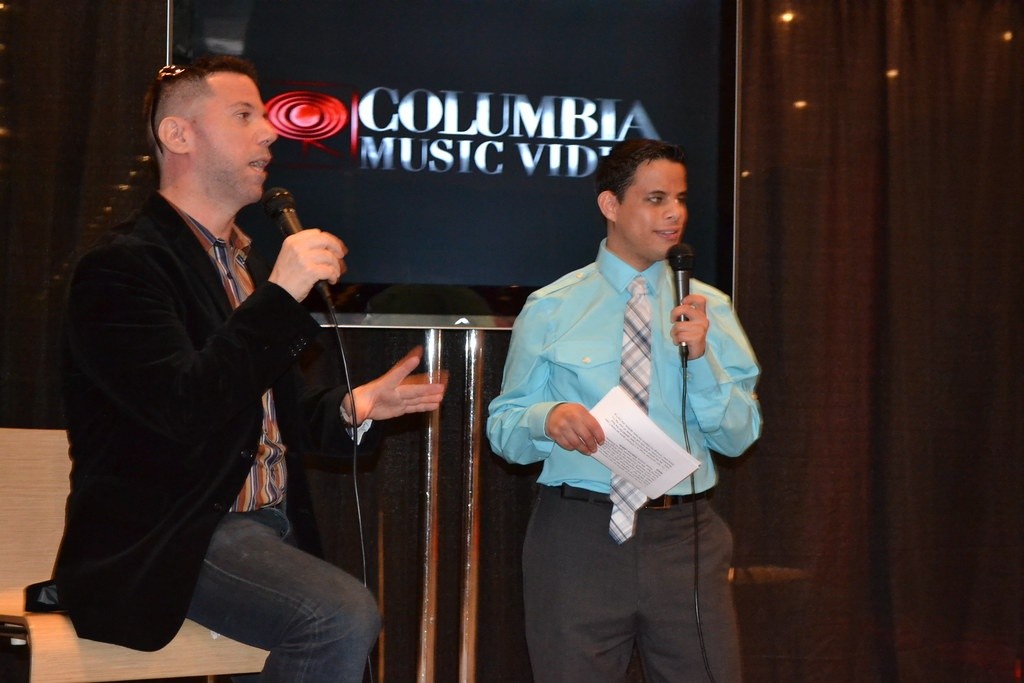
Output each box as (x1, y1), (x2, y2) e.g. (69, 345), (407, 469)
(664, 242), (693, 366)
(261, 187), (332, 305)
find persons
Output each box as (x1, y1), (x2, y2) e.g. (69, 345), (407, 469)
(487, 138), (764, 683)
(24, 54), (444, 683)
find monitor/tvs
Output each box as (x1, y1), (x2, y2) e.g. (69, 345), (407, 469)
(167, 0), (744, 328)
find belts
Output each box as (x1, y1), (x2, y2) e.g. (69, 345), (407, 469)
(559, 482), (705, 509)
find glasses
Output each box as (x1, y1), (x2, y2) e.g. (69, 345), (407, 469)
(150, 63), (192, 155)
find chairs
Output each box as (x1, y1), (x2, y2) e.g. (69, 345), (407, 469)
(0, 429), (272, 683)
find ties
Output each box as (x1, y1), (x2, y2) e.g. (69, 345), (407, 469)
(609, 276), (652, 546)
